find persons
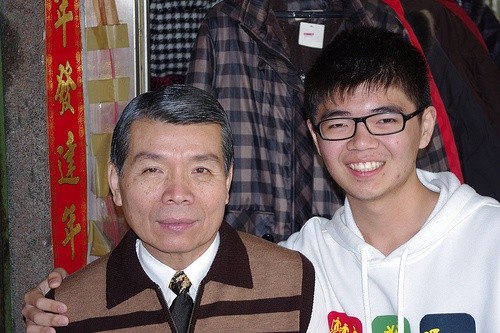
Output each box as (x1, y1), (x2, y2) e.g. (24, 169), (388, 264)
(21, 35), (499, 332)
(37, 81), (319, 333)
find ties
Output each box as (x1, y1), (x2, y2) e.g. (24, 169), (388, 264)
(169, 271), (193, 333)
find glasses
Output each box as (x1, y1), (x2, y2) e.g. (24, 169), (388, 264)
(314, 109), (422, 141)
